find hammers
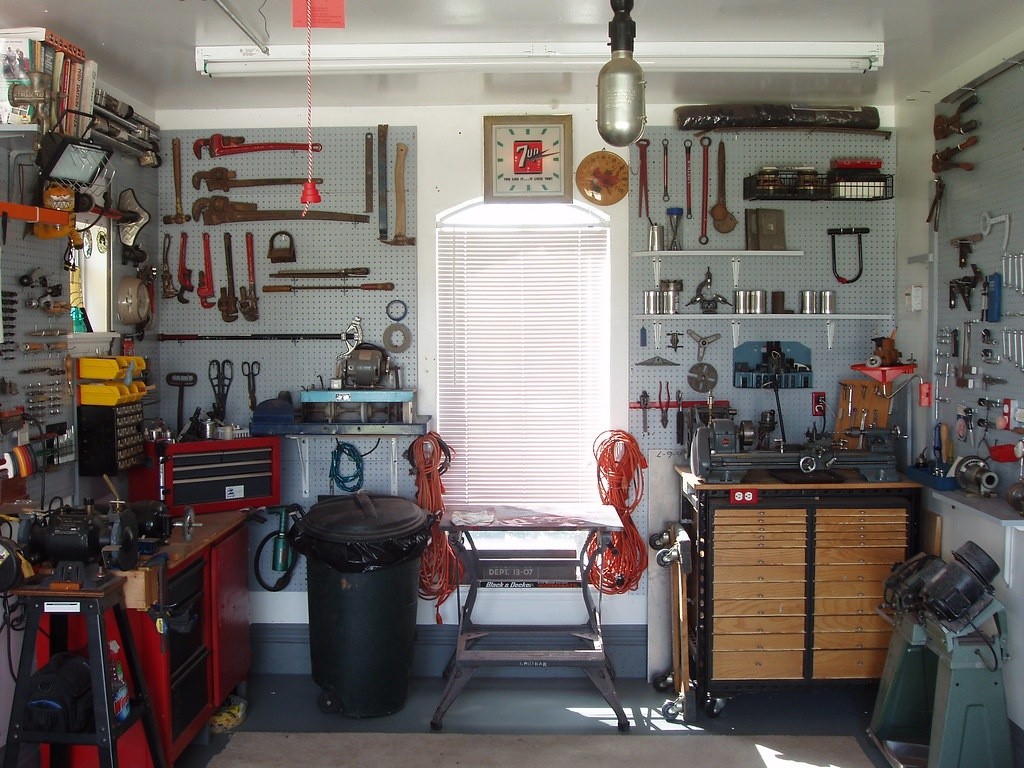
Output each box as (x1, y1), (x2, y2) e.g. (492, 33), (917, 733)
(380, 142), (417, 246)
(162, 137), (191, 224)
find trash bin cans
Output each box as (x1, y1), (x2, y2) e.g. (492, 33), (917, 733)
(287, 489), (441, 722)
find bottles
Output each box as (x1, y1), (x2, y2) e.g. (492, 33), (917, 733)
(108, 658), (133, 724)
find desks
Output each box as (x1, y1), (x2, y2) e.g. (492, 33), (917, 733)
(429, 504), (630, 732)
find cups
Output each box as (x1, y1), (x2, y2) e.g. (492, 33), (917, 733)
(665, 207), (684, 251)
(647, 224), (664, 251)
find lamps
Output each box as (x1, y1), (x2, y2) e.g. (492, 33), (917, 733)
(35, 109), (114, 189)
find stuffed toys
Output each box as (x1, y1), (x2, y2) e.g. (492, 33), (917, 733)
(32, 183), (86, 250)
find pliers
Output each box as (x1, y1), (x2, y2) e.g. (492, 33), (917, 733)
(241, 361), (261, 410)
(656, 380), (671, 428)
(207, 359), (234, 423)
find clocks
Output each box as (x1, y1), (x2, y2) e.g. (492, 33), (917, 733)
(483, 114), (573, 204)
(575, 151), (629, 206)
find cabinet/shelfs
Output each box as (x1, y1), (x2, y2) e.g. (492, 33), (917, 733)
(674, 463), (923, 717)
(27, 510), (250, 768)
(249, 387), (433, 497)
(1, 88), (162, 169)
(629, 125), (898, 596)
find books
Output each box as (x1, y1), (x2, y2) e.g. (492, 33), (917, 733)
(0, 26), (99, 141)
(742, 207), (788, 251)
